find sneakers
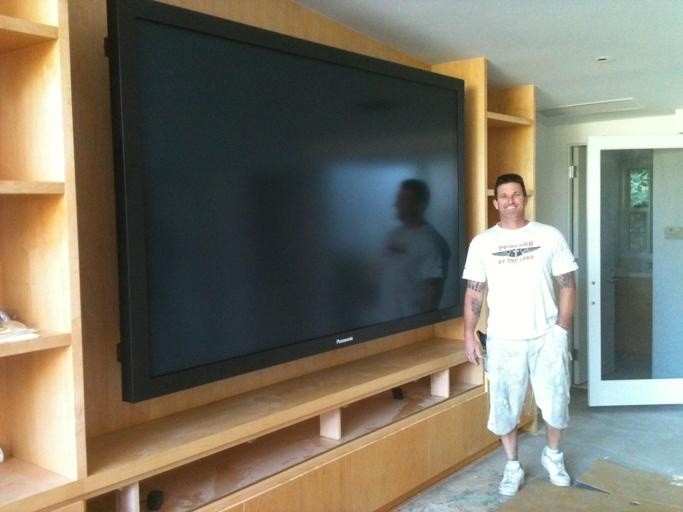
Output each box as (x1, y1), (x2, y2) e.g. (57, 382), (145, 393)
(500, 466), (524, 496)
(540, 447), (571, 487)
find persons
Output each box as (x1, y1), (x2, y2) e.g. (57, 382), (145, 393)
(366, 177), (453, 324)
(459, 171), (581, 499)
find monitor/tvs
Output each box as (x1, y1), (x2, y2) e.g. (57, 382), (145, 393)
(108, 0), (465, 404)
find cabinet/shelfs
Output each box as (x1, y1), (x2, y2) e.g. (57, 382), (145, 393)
(0, 0), (79, 511)
(85, 337), (538, 511)
(486, 55), (534, 231)
